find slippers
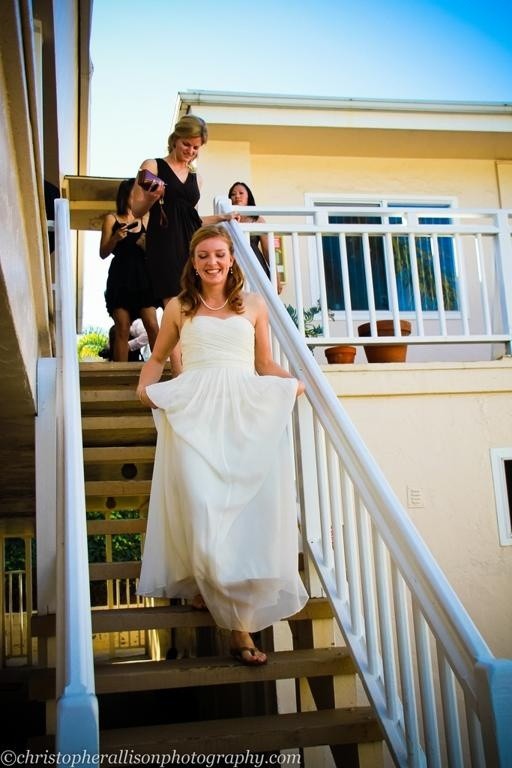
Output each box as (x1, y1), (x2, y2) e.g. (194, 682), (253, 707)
(226, 644), (270, 667)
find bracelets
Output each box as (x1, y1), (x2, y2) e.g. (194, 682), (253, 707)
(138, 387), (146, 405)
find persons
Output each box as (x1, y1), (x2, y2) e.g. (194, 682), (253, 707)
(99, 177), (161, 362)
(223, 179), (283, 296)
(135, 225), (310, 666)
(97, 321), (150, 361)
(131, 113), (241, 381)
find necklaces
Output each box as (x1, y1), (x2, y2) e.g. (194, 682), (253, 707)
(197, 289), (233, 311)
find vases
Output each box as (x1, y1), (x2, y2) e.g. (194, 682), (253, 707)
(358, 319), (412, 362)
(324, 345), (356, 363)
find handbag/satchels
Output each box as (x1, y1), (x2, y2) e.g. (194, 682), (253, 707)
(137, 165), (168, 229)
